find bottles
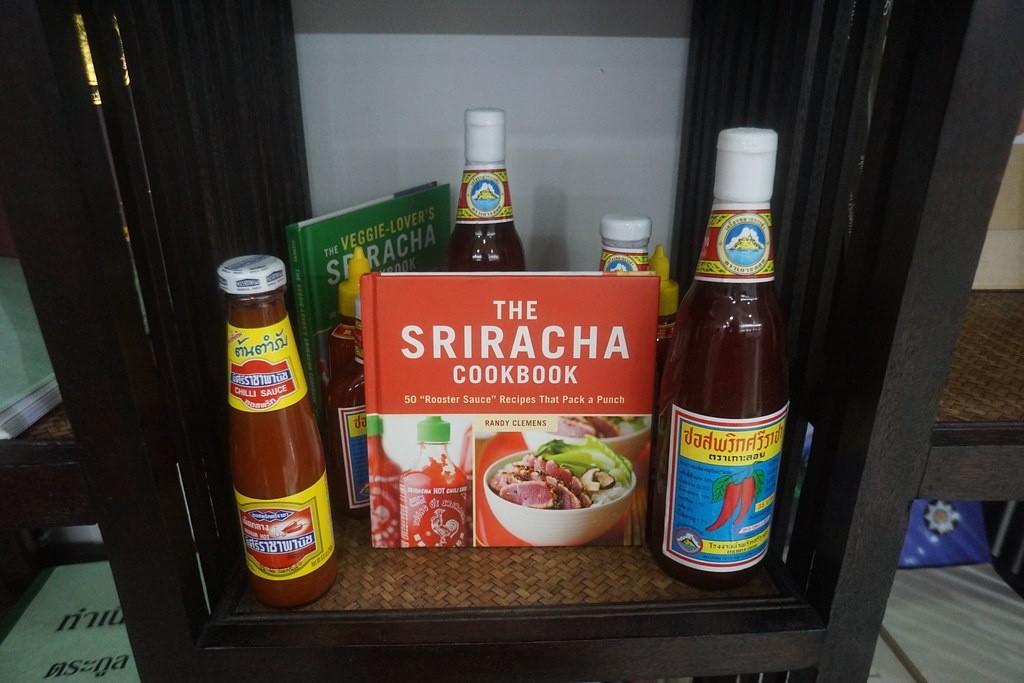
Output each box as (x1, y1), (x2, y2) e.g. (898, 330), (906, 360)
(217, 254), (339, 610)
(446, 108), (526, 273)
(325, 241), (380, 518)
(595, 211), (678, 416)
(369, 415), (467, 547)
(646, 127), (791, 590)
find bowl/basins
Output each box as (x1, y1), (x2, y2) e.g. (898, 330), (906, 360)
(536, 411), (655, 472)
(481, 450), (638, 550)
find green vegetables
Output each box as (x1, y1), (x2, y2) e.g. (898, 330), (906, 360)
(530, 434), (633, 488)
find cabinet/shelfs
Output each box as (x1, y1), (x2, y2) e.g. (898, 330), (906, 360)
(0, 0), (1024, 683)
